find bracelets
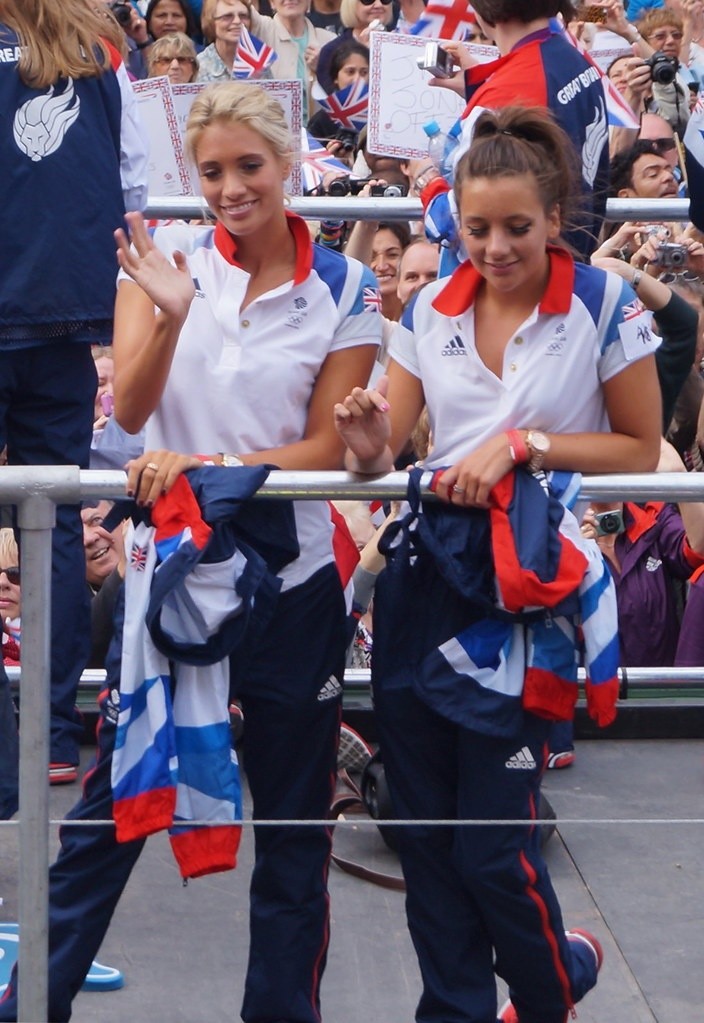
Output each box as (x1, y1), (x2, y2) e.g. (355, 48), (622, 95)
(361, 219), (378, 232)
(414, 167), (438, 194)
(193, 454), (215, 467)
(502, 428), (527, 463)
(630, 267), (641, 288)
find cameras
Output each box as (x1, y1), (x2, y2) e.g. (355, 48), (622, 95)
(639, 225), (672, 245)
(417, 41), (454, 79)
(652, 243), (687, 269)
(330, 177), (379, 197)
(637, 51), (680, 85)
(577, 5), (607, 24)
(594, 509), (625, 537)
(371, 185), (407, 198)
(336, 126), (359, 152)
(106, 0), (131, 27)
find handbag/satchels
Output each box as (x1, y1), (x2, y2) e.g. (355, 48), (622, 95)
(329, 755), (559, 886)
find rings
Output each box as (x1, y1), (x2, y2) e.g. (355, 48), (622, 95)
(580, 526), (585, 532)
(147, 463), (158, 470)
(452, 484), (464, 495)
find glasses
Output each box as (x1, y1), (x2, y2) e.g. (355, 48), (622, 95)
(646, 32), (683, 40)
(154, 55), (197, 65)
(0, 566), (21, 586)
(358, 0), (393, 6)
(214, 12), (254, 23)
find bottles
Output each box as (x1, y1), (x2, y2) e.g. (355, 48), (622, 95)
(422, 121), (461, 189)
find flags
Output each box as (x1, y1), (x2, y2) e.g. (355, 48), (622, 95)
(409, 0), (477, 42)
(555, 18), (639, 128)
(315, 75), (369, 131)
(231, 25), (277, 80)
(301, 125), (352, 191)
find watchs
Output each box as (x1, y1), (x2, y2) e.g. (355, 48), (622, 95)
(219, 452), (243, 467)
(525, 428), (551, 473)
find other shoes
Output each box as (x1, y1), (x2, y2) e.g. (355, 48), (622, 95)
(499, 928), (603, 1023)
(42, 759), (79, 786)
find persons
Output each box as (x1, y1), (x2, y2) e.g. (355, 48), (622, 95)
(0, 0), (704, 822)
(333, 104), (663, 1023)
(0, 82), (381, 1023)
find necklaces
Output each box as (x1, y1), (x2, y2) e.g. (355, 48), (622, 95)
(691, 35), (702, 43)
(598, 539), (616, 548)
